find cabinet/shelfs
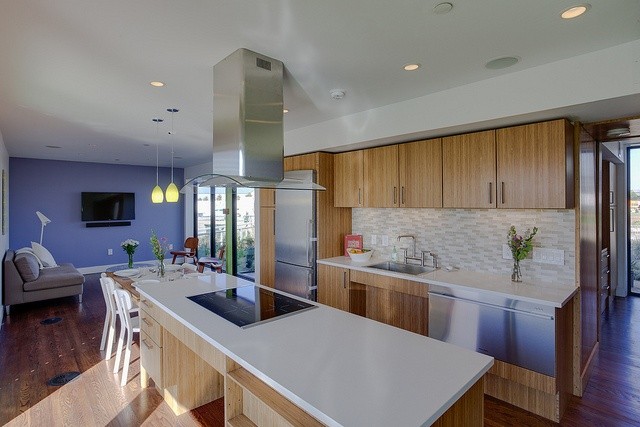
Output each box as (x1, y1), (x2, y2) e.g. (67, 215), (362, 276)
(333, 149), (364, 208)
(600, 247), (610, 325)
(363, 137), (443, 208)
(602, 205), (618, 292)
(225, 357), (332, 427)
(140, 292), (224, 417)
(442, 119), (575, 209)
(602, 161), (617, 205)
(317, 263), (366, 317)
(258, 150), (352, 289)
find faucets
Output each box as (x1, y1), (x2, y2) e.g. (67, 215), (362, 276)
(397, 235), (416, 258)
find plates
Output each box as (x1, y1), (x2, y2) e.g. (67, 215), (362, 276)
(130, 279), (162, 288)
(113, 270), (142, 277)
(159, 264), (183, 273)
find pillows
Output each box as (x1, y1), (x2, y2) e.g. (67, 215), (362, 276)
(30, 241), (57, 267)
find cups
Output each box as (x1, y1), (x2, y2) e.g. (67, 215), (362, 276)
(210, 272), (216, 291)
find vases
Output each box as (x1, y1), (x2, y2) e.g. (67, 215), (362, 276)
(157, 262), (165, 277)
(128, 259), (133, 268)
(510, 261), (522, 282)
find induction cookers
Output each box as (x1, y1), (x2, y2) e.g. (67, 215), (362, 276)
(188, 281), (316, 330)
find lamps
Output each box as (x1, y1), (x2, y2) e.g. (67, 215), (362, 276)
(165, 107), (180, 203)
(35, 210), (51, 246)
(151, 117), (164, 204)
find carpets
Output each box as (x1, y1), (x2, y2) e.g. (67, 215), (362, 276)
(190, 396), (224, 427)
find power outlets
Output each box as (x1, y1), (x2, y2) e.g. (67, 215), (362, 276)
(502, 244), (513, 259)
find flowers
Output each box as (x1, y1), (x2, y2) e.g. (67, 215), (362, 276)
(149, 230), (173, 277)
(120, 238), (139, 268)
(506, 224), (539, 281)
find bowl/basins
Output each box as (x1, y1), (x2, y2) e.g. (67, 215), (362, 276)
(347, 247), (373, 264)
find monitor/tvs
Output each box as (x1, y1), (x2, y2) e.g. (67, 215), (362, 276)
(82, 191), (136, 221)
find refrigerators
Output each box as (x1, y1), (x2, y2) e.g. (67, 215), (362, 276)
(273, 169), (317, 301)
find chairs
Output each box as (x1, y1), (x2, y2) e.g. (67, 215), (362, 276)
(198, 244), (225, 273)
(170, 237), (199, 265)
(113, 288), (140, 387)
(99, 272), (123, 360)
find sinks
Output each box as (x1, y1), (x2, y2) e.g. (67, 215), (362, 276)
(369, 260), (433, 275)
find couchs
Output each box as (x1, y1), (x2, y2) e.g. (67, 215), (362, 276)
(2, 247), (85, 315)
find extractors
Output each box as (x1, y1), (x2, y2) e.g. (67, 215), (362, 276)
(182, 47), (328, 204)
